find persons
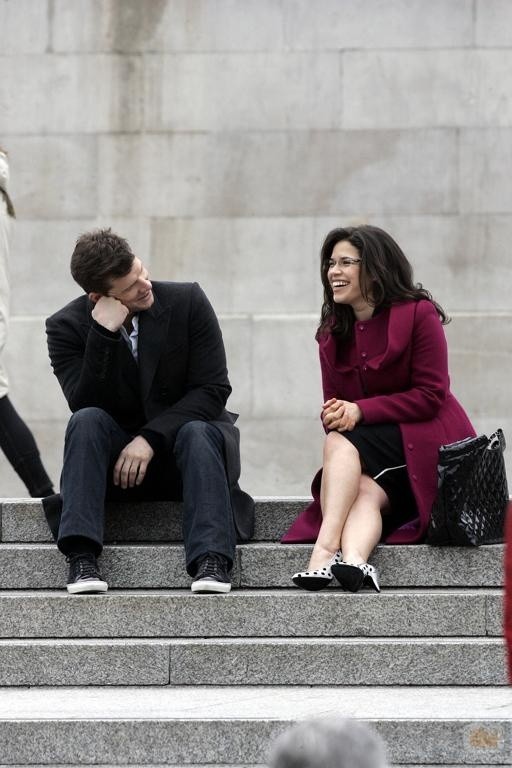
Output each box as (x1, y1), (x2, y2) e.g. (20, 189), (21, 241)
(1, 142), (57, 499)
(291, 222), (493, 592)
(44, 232), (242, 593)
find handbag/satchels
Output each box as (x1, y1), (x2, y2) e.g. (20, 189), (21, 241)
(427, 428), (507, 546)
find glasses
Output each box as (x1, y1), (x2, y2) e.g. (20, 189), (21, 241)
(329, 257), (361, 267)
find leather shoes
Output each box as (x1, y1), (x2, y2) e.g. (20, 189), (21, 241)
(291, 549), (344, 592)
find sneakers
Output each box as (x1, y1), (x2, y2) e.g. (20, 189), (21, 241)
(191, 552), (232, 594)
(67, 550), (109, 593)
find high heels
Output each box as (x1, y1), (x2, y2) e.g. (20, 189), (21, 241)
(331, 560), (382, 594)
